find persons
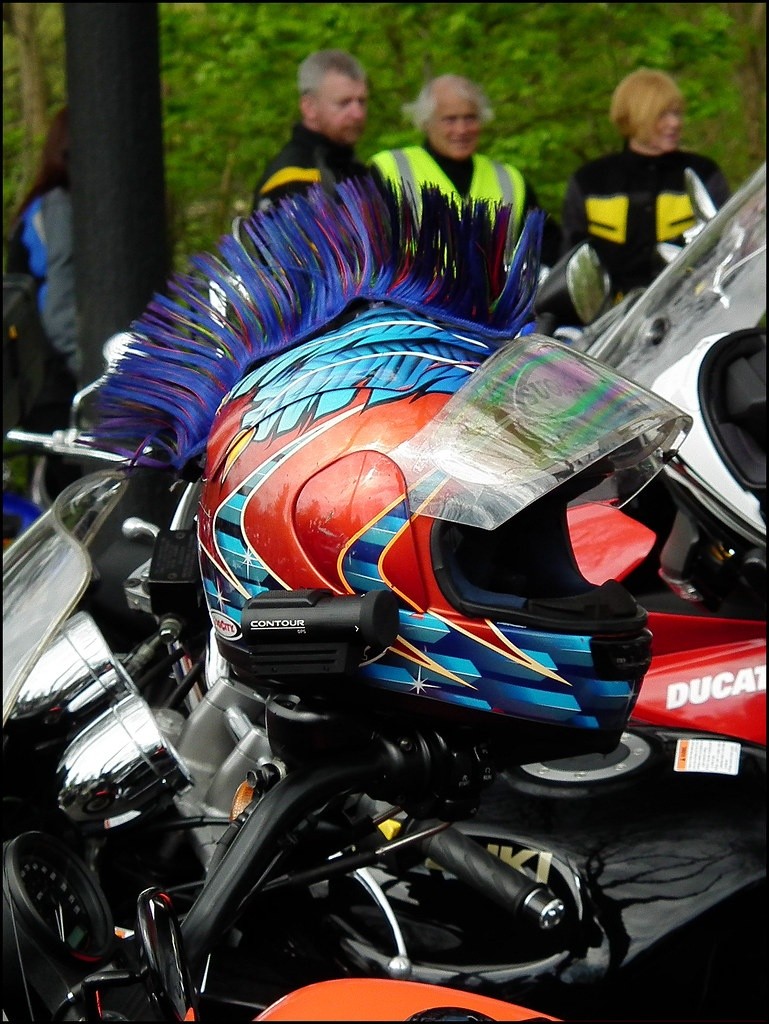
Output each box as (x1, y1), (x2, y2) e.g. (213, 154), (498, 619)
(367, 74), (561, 314)
(252, 49), (366, 265)
(6, 105), (79, 373)
(563, 68), (731, 306)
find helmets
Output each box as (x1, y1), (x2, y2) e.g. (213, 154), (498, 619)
(196, 303), (695, 757)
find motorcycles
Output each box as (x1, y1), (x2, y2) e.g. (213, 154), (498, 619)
(1, 162), (766, 1024)
